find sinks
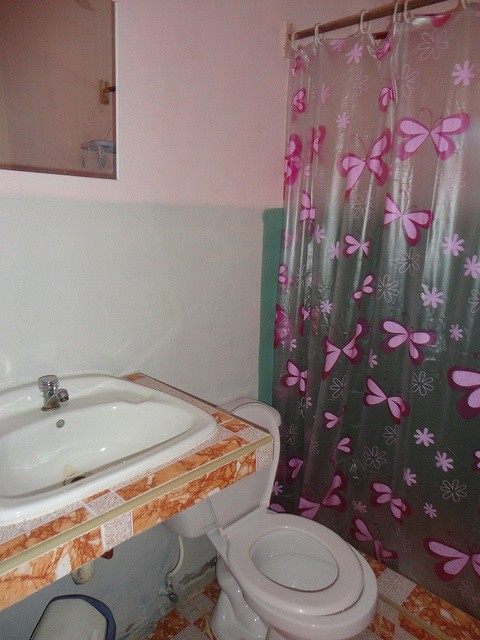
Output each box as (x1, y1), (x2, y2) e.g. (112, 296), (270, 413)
(1, 397), (197, 505)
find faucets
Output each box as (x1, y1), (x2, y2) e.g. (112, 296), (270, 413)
(37, 372), (70, 413)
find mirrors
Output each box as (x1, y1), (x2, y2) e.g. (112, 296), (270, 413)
(0, 0), (117, 181)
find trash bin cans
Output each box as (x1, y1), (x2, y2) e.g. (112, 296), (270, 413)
(29, 594), (116, 640)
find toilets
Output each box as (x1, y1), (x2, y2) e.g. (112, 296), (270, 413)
(160, 394), (382, 640)
